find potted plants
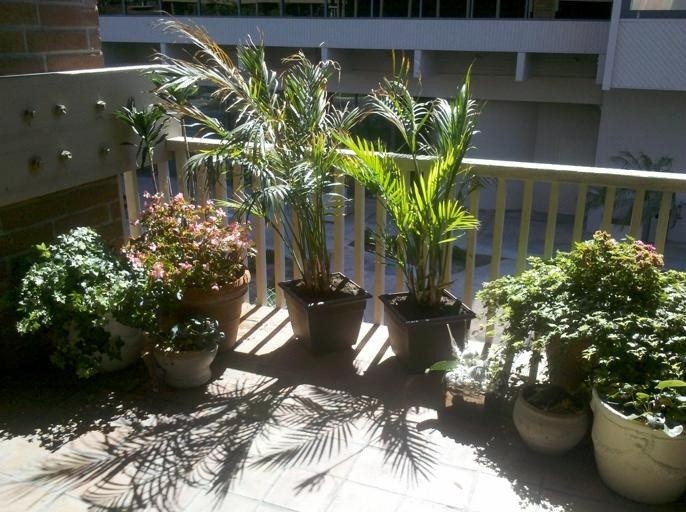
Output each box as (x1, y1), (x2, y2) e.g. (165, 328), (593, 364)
(424, 321), (506, 422)
(573, 229), (686, 508)
(316, 55), (487, 375)
(110, 9), (390, 358)
(18, 223), (154, 376)
(477, 241), (592, 457)
